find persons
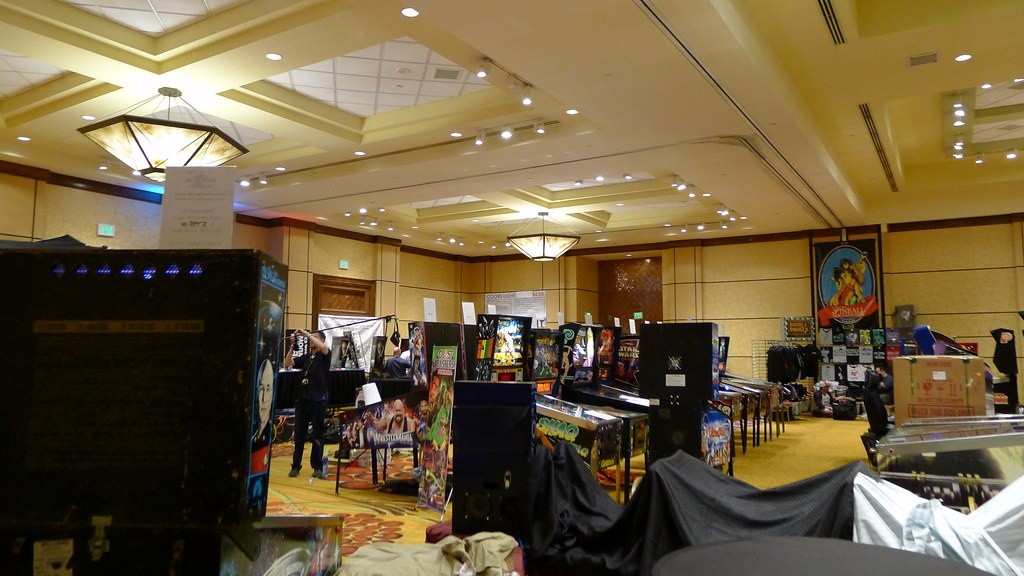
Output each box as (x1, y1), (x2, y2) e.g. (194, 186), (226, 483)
(811, 381), (850, 418)
(340, 340), (351, 366)
(342, 394), (430, 447)
(375, 340), (385, 368)
(582, 326), (595, 368)
(497, 328), (517, 365)
(253, 358), (273, 450)
(383, 346), (412, 378)
(410, 327), (426, 386)
(860, 362), (895, 422)
(283, 327), (332, 478)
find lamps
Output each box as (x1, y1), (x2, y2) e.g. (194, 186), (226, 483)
(506, 211), (580, 262)
(76, 88), (249, 184)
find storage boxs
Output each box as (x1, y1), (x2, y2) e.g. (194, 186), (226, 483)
(891, 355), (986, 429)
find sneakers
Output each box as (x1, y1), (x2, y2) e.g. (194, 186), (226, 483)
(289, 468), (299, 477)
(312, 469), (322, 479)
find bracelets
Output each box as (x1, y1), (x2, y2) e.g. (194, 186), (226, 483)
(307, 332), (312, 338)
(290, 345), (294, 349)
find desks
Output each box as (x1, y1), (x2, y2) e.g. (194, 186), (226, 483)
(274, 368), (367, 408)
(373, 378), (412, 402)
(652, 536), (996, 576)
